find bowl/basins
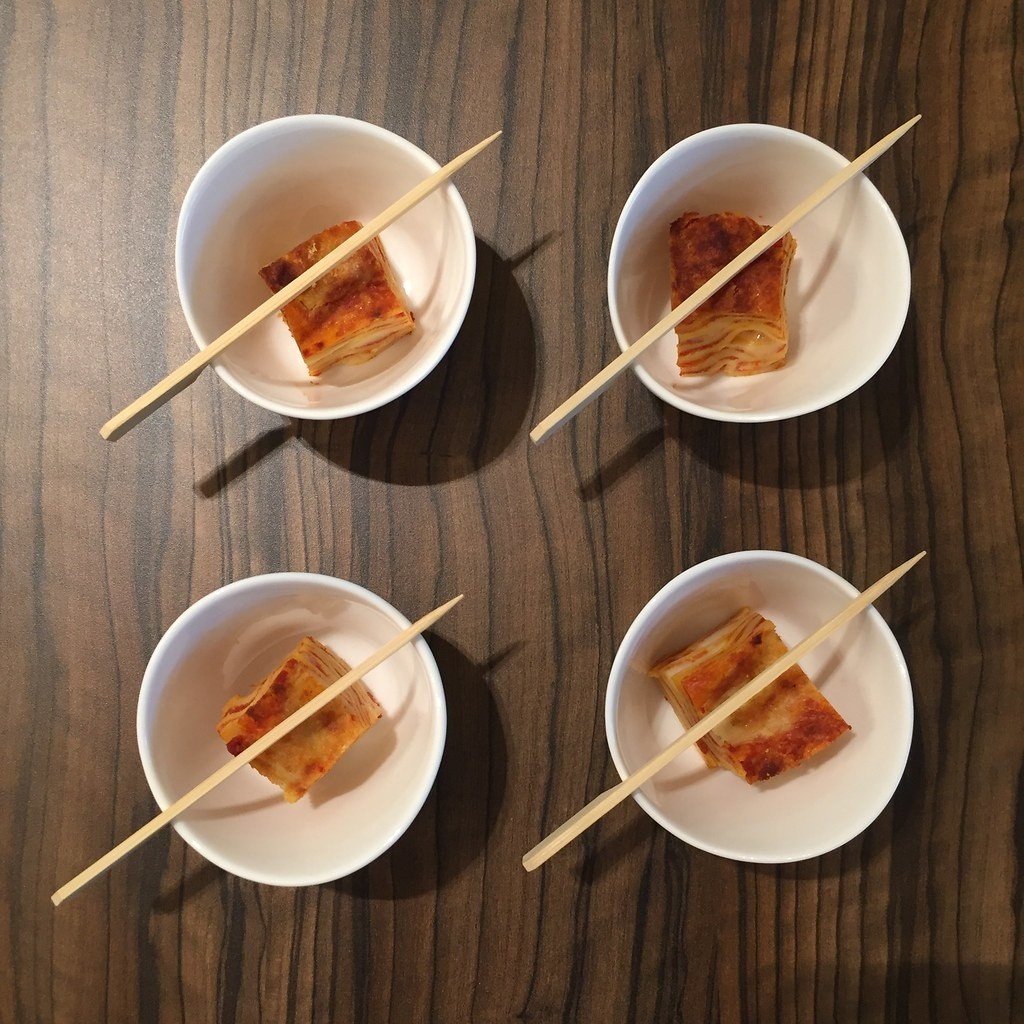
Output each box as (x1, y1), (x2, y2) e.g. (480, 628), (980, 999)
(136, 572), (447, 887)
(604, 551), (914, 864)
(175, 114), (476, 420)
(607, 124), (911, 423)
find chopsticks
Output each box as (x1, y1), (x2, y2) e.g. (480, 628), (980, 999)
(42, 85), (929, 912)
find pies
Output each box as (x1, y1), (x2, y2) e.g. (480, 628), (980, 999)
(648, 612), (851, 786)
(258, 222), (416, 373)
(668, 218), (791, 375)
(221, 641), (382, 805)
